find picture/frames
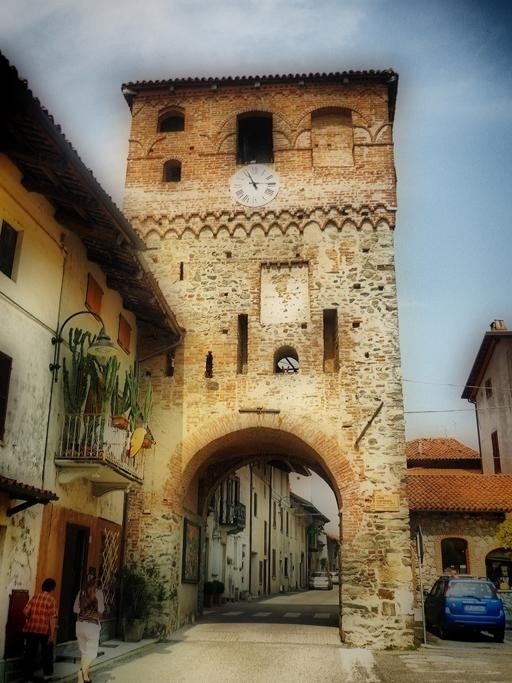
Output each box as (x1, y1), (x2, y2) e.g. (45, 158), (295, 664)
(181, 516), (201, 584)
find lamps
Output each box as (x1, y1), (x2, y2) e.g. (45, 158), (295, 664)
(49, 309), (119, 383)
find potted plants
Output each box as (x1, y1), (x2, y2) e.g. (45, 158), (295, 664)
(119, 558), (177, 642)
(63, 327), (156, 462)
(213, 579), (225, 604)
(204, 581), (214, 608)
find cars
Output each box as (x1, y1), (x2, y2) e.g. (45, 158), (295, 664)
(309, 571), (335, 590)
(330, 570), (339, 584)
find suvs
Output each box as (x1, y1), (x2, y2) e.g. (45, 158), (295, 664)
(423, 572), (506, 644)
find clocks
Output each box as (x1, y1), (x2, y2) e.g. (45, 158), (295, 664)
(230, 164), (281, 207)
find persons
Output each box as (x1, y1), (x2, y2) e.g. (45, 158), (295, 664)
(21, 576), (59, 682)
(72, 577), (107, 682)
